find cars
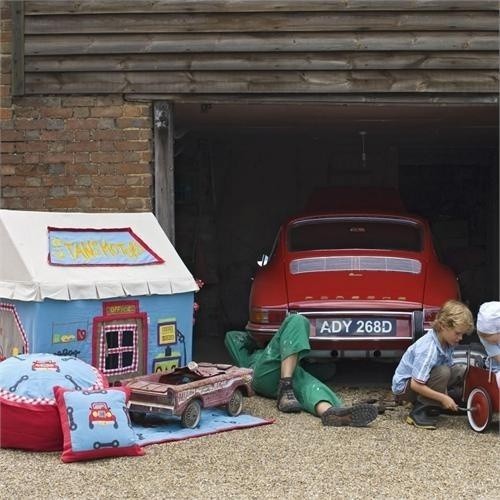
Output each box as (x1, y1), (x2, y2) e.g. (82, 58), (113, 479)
(247, 212), (462, 361)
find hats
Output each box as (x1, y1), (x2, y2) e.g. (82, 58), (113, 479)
(477, 301), (500, 334)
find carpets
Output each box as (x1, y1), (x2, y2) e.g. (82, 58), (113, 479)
(134, 396), (274, 448)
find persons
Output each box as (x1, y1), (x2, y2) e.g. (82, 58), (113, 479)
(475, 300), (500, 390)
(392, 298), (475, 429)
(222, 313), (379, 426)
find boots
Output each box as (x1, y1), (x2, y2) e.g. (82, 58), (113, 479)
(407, 401), (436, 430)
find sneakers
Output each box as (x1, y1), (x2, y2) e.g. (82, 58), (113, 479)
(276, 386), (299, 411)
(321, 403), (378, 428)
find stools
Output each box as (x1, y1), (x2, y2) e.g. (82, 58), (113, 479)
(1, 352), (109, 453)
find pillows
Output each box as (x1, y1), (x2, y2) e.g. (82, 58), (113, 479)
(53, 384), (145, 462)
